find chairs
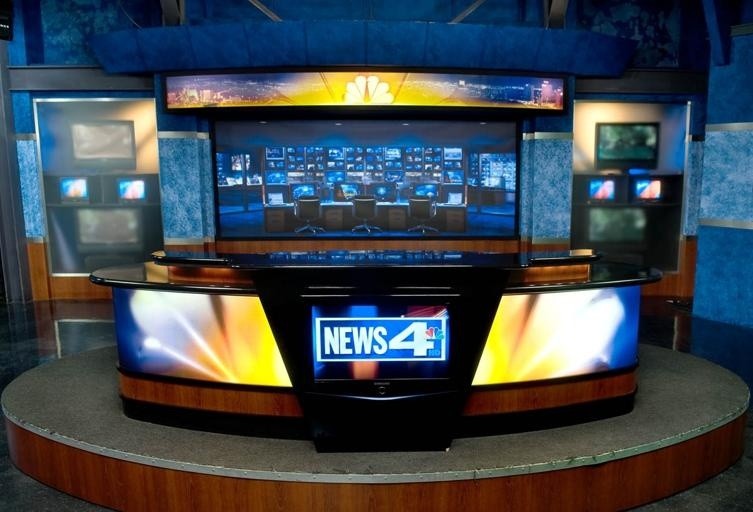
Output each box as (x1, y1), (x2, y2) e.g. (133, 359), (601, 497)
(293, 198), (441, 236)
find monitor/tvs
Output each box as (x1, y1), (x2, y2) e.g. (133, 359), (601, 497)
(583, 122), (666, 253)
(58, 119), (150, 253)
(300, 294), (463, 401)
(217, 143), (518, 206)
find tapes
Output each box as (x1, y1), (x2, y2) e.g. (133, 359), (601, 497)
(569, 249), (592, 256)
